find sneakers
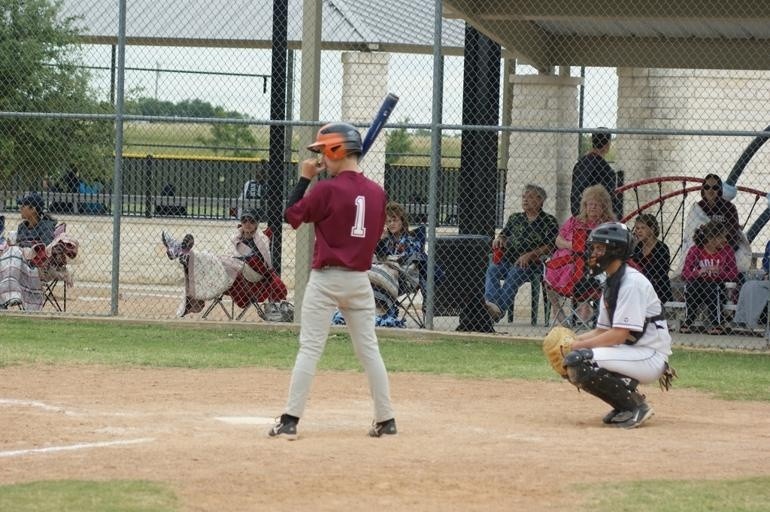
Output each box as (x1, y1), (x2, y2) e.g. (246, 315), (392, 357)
(269, 414), (299, 439)
(180, 235), (194, 264)
(370, 420), (397, 438)
(603, 404), (655, 428)
(161, 231), (180, 258)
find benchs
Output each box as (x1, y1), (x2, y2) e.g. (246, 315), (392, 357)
(660, 250), (770, 336)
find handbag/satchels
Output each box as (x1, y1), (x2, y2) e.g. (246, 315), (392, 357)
(457, 293), (497, 330)
(257, 301), (294, 322)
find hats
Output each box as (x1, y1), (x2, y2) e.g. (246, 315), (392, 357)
(18, 194), (44, 208)
(240, 209), (258, 222)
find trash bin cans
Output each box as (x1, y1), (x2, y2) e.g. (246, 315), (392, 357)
(433, 235), (493, 316)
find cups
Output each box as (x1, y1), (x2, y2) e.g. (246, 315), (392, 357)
(493, 247), (502, 263)
(726, 282), (737, 305)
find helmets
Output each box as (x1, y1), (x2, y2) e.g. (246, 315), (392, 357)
(586, 221), (634, 276)
(307, 123), (363, 162)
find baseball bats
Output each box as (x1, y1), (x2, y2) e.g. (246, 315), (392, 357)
(358, 94), (400, 166)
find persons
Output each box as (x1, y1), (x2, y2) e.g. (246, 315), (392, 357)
(540, 218), (679, 429)
(34, 160), (110, 218)
(235, 173), (264, 216)
(331, 202), (425, 326)
(570, 127), (617, 219)
(161, 209), (287, 317)
(679, 173), (768, 337)
(16, 193), (57, 270)
(266, 120), (400, 440)
(484, 184), (675, 323)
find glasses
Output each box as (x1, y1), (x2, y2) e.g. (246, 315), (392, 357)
(703, 184), (720, 191)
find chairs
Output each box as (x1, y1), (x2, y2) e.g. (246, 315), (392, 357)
(484, 247), (551, 326)
(4, 222), (68, 313)
(369, 221), (428, 329)
(202, 227), (275, 321)
(544, 224), (604, 335)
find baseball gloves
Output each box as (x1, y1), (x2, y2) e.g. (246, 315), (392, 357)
(544, 324), (577, 379)
